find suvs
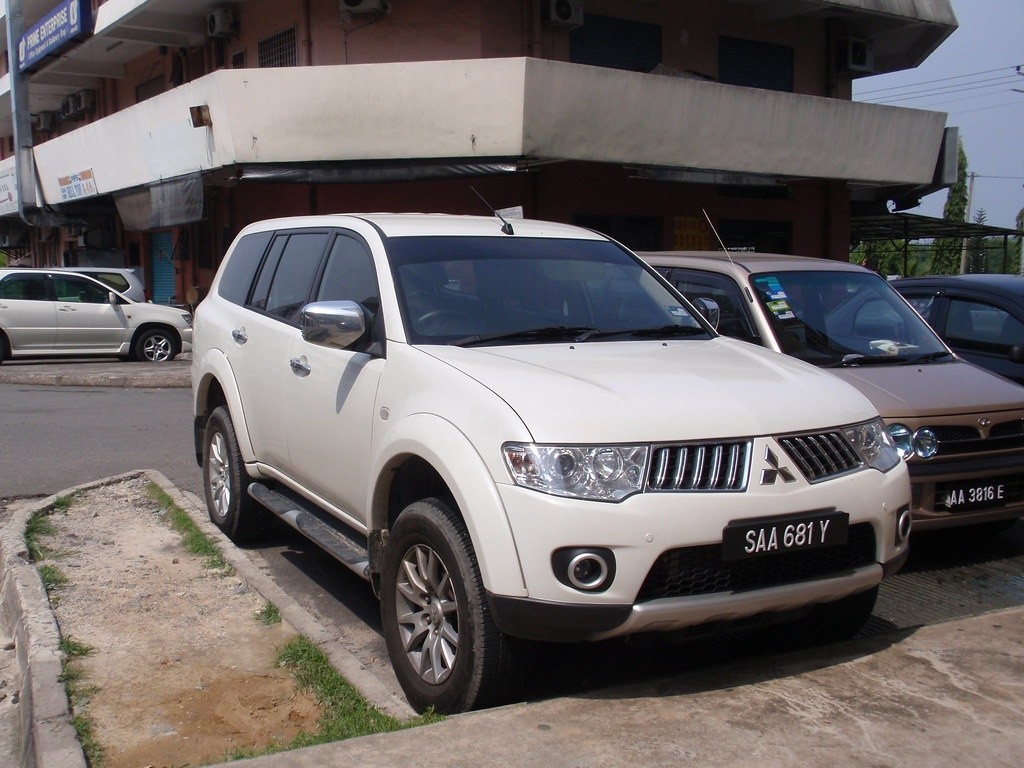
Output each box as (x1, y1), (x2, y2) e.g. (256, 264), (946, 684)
(45, 268), (146, 303)
(540, 250), (1024, 529)
(189, 213), (914, 716)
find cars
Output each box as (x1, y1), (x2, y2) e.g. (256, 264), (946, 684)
(0, 269), (193, 363)
(824, 273), (1024, 385)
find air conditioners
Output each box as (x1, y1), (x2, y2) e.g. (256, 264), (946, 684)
(27, 214), (39, 225)
(338, 0), (385, 17)
(59, 90), (93, 119)
(2, 232), (18, 247)
(543, 0), (585, 30)
(840, 35), (875, 71)
(206, 6), (234, 37)
(36, 113), (53, 132)
(66, 224), (82, 237)
